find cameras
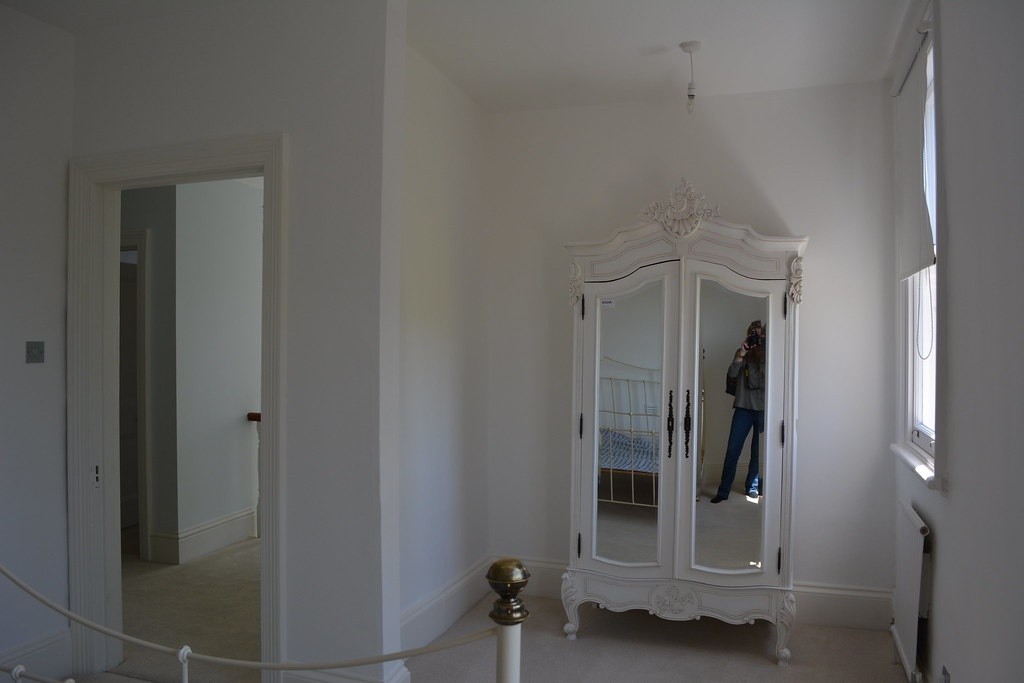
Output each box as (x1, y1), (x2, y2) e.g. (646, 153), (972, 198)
(747, 329), (760, 346)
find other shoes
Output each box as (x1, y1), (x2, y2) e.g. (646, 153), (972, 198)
(747, 492), (758, 498)
(711, 494), (728, 503)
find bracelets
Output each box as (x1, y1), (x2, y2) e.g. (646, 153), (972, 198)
(738, 354), (744, 359)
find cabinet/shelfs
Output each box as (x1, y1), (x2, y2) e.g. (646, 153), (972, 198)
(562, 176), (810, 669)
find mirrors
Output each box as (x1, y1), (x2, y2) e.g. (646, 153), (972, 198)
(692, 276), (772, 570)
(595, 273), (668, 566)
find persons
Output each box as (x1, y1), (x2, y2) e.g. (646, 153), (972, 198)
(710, 321), (768, 504)
